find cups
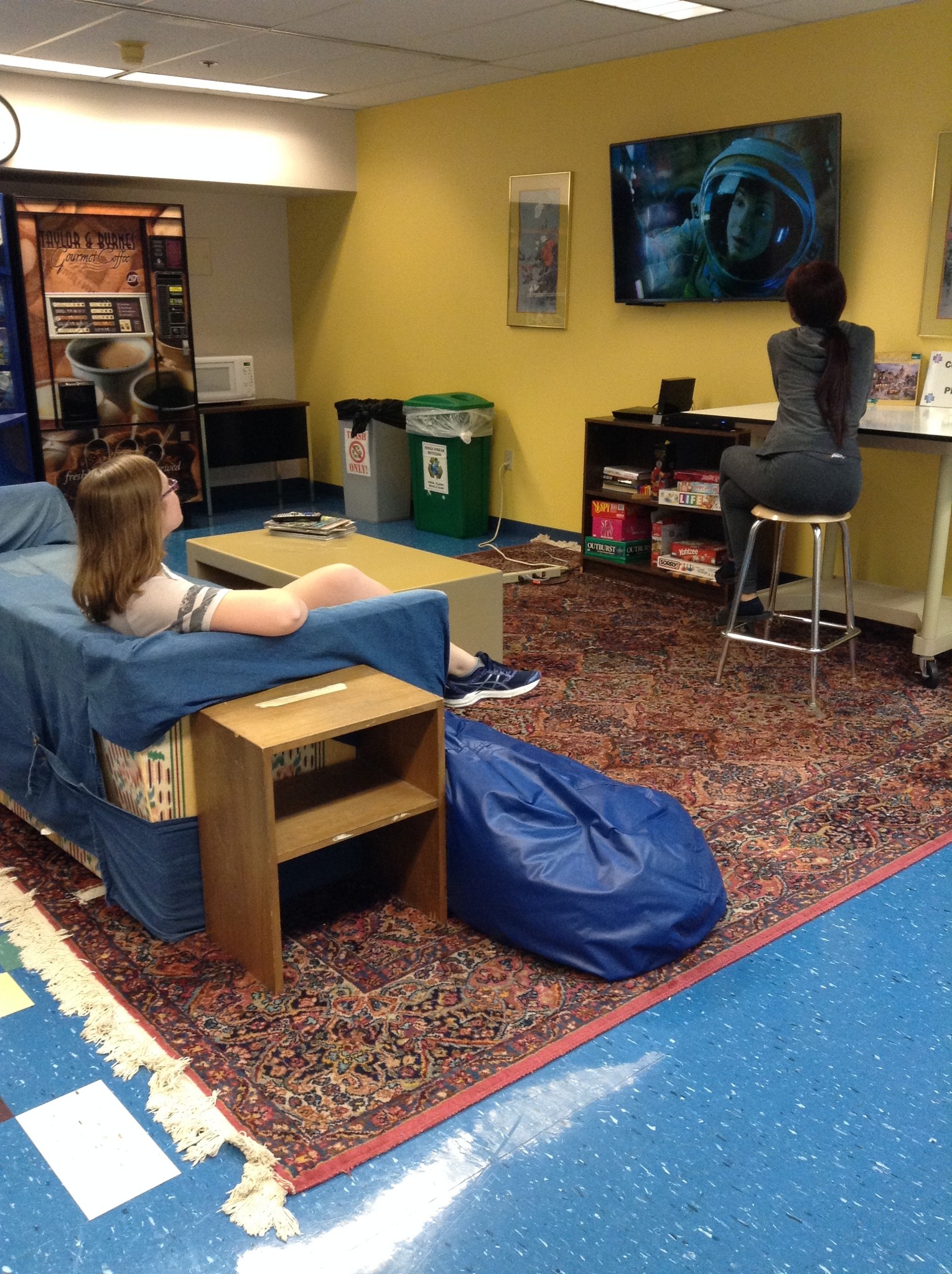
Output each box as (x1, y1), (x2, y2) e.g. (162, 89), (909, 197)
(35, 378), (104, 428)
(65, 337), (152, 399)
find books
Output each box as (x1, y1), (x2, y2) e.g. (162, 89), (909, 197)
(263, 510), (358, 541)
(602, 464), (653, 494)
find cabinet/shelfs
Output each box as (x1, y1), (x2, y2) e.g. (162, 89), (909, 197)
(578, 416), (752, 606)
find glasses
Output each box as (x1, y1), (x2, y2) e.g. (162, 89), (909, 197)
(162, 477), (179, 498)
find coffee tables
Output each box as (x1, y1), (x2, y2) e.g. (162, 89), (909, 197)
(185, 527), (505, 666)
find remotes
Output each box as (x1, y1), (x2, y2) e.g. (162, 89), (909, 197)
(271, 512), (323, 520)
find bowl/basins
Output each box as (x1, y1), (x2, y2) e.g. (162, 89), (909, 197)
(156, 337), (192, 369)
(131, 368), (197, 427)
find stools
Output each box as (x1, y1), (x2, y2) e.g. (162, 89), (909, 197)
(712, 504), (863, 711)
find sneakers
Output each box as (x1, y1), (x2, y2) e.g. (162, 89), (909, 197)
(443, 651), (541, 709)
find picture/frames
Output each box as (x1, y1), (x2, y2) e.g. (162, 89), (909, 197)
(866, 350), (922, 406)
(506, 171), (575, 330)
(916, 130), (952, 339)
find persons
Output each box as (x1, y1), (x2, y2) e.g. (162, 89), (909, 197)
(70, 454), (542, 709)
(713, 260), (876, 626)
(647, 136), (819, 300)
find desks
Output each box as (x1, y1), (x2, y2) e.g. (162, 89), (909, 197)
(681, 401), (952, 693)
(198, 397), (315, 517)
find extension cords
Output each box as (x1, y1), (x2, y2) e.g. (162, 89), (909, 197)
(503, 566), (563, 583)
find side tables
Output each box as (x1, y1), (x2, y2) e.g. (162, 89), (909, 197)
(192, 664), (449, 999)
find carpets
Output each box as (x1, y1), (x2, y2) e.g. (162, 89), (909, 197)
(0, 531), (952, 1244)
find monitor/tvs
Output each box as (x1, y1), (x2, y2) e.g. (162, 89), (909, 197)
(609, 112), (844, 303)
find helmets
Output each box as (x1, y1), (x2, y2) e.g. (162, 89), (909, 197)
(696, 137), (814, 295)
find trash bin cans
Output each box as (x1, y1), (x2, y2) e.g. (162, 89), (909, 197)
(402, 392), (495, 539)
(334, 399), (412, 523)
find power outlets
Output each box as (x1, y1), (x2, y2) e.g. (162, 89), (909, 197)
(504, 449), (513, 471)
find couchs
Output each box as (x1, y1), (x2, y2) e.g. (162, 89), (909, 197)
(0, 478), (448, 947)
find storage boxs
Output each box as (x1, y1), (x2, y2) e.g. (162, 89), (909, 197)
(650, 510), (688, 568)
(656, 554), (724, 580)
(677, 480), (720, 495)
(584, 535), (652, 564)
(592, 517), (653, 542)
(658, 487), (723, 512)
(669, 539), (728, 567)
(591, 499), (658, 520)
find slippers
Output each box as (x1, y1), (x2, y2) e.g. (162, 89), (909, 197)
(711, 605), (774, 626)
(715, 560), (737, 585)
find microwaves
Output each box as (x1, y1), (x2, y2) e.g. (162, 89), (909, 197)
(194, 355), (257, 404)
